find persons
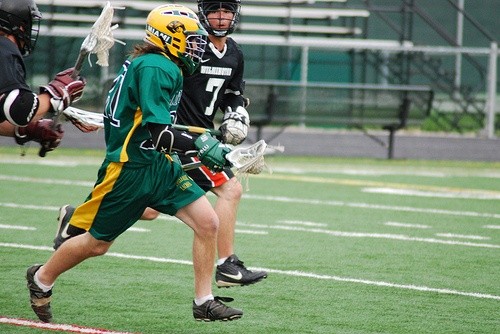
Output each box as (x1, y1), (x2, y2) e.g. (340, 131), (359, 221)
(25, 3), (244, 323)
(0, 0), (86, 151)
(54, 0), (269, 289)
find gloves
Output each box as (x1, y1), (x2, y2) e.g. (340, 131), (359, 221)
(216, 106), (250, 145)
(195, 132), (232, 172)
(39, 68), (86, 115)
(14, 119), (64, 152)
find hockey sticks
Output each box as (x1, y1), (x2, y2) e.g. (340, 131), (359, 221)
(180, 139), (266, 175)
(39, 3), (114, 157)
(63, 106), (220, 137)
(182, 130), (287, 157)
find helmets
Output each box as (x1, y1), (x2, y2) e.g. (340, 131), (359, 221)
(144, 4), (209, 76)
(0, 1), (41, 57)
(198, 0), (241, 36)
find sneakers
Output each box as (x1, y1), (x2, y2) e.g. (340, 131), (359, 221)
(26, 265), (53, 323)
(216, 255), (267, 288)
(193, 296), (244, 321)
(54, 205), (75, 254)
